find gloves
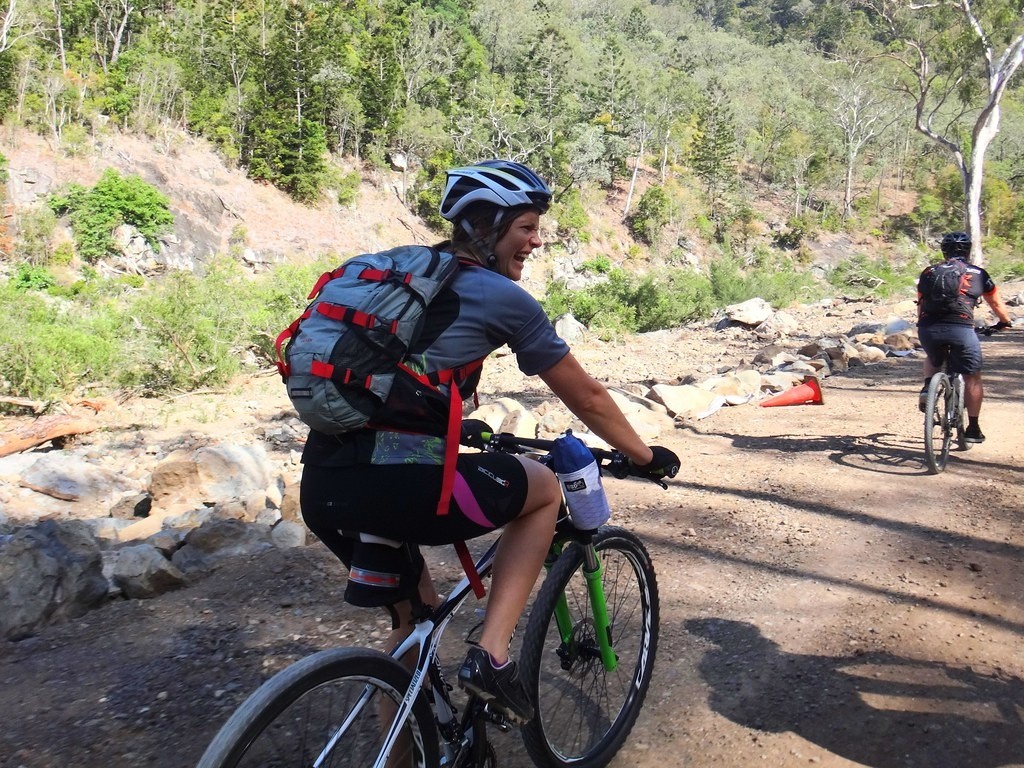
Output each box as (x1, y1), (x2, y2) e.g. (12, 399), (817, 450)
(461, 419), (493, 451)
(632, 445), (680, 479)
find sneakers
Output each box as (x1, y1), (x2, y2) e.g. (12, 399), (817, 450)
(458, 645), (534, 726)
(919, 387), (929, 412)
(963, 427), (985, 443)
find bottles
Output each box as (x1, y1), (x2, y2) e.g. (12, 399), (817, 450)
(552, 434), (609, 530)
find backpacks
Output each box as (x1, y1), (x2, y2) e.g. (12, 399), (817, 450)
(918, 259), (968, 303)
(276, 240), (490, 437)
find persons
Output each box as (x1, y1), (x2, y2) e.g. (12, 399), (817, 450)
(299, 160), (681, 768)
(917, 231), (1014, 442)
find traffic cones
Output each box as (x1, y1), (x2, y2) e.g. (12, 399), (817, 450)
(760, 373), (826, 408)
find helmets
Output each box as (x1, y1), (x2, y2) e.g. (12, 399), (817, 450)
(942, 231), (971, 254)
(440, 159), (551, 220)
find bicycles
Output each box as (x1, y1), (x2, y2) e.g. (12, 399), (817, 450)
(922, 322), (1012, 476)
(194, 428), (677, 768)
